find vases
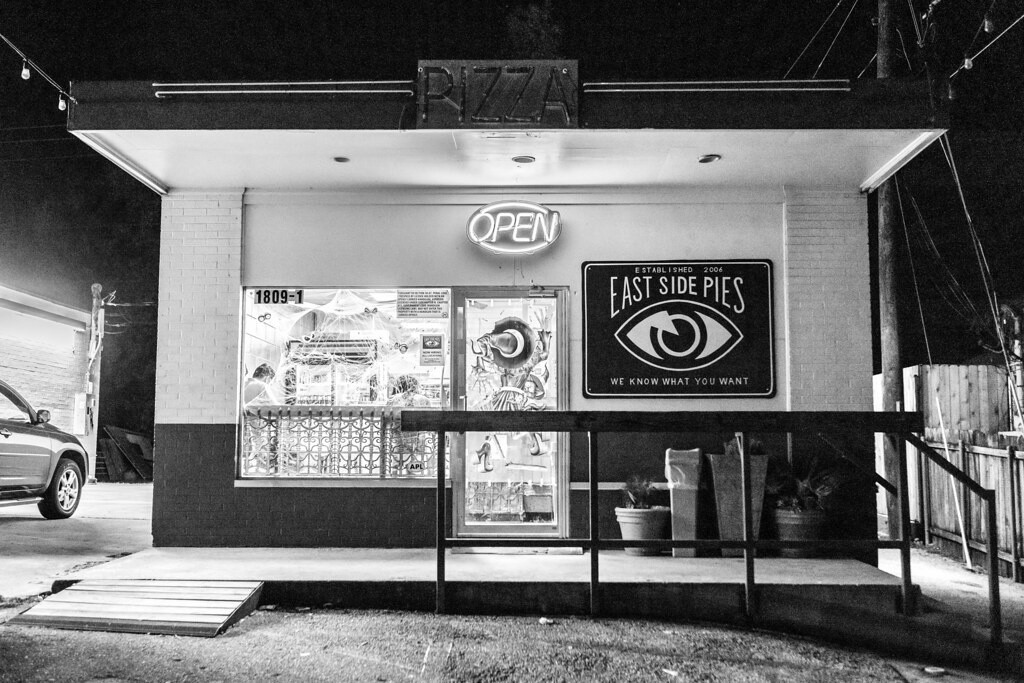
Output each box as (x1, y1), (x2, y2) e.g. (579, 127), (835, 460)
(665, 448), (702, 559)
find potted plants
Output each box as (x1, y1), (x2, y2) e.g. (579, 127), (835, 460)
(614, 475), (670, 557)
(764, 455), (847, 559)
(707, 437), (770, 555)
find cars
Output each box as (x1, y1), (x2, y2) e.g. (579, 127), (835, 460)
(0, 378), (89, 518)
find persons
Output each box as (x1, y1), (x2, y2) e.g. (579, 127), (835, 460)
(383, 375), (431, 453)
(244, 363), (280, 405)
